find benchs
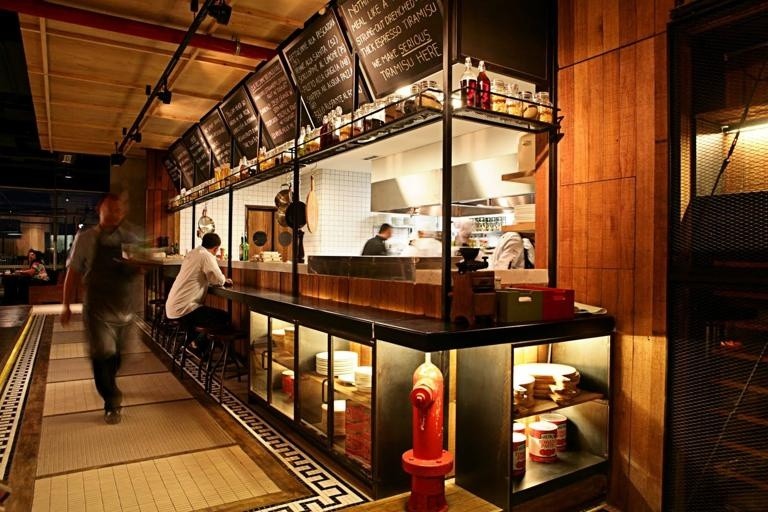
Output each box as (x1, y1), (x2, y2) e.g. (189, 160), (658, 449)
(28, 262), (77, 304)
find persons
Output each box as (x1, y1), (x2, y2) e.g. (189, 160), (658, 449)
(60, 192), (162, 424)
(13, 248), (50, 305)
(488, 231), (525, 270)
(362, 223), (391, 255)
(521, 233), (536, 269)
(165, 231), (237, 364)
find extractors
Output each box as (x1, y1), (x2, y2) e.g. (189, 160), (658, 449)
(370, 151), (535, 216)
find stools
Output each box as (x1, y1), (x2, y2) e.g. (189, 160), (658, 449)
(148, 296), (246, 407)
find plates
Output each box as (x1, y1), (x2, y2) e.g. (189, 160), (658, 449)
(320, 399), (346, 431)
(353, 364), (373, 394)
(315, 350), (360, 377)
(514, 364), (582, 409)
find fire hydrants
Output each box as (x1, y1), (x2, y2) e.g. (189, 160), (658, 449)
(400, 361), (453, 512)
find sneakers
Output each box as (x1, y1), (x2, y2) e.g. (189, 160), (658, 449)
(103, 404), (122, 426)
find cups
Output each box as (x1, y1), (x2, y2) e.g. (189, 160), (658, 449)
(513, 414), (569, 477)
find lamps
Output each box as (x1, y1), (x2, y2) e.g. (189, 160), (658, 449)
(106, 1), (237, 168)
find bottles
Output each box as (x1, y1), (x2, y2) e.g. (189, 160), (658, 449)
(170, 239), (179, 254)
(169, 77), (440, 214)
(470, 214), (508, 231)
(239, 232), (250, 261)
(466, 234), (488, 247)
(458, 56), (492, 110)
(488, 78), (553, 124)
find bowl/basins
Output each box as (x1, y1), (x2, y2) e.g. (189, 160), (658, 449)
(270, 328), (294, 355)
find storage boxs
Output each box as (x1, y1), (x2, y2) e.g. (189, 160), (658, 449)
(505, 285), (577, 321)
(496, 287), (544, 325)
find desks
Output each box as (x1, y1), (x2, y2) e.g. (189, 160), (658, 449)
(0, 274), (35, 304)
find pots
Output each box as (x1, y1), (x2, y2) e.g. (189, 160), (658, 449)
(273, 181), (306, 228)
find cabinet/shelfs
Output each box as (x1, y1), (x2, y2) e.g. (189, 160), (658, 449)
(295, 318), (440, 490)
(246, 301), (329, 432)
(457, 332), (613, 503)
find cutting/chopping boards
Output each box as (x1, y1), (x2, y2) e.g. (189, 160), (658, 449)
(306, 173), (322, 233)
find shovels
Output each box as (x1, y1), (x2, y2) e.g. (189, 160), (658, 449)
(690, 345), (767, 501)
